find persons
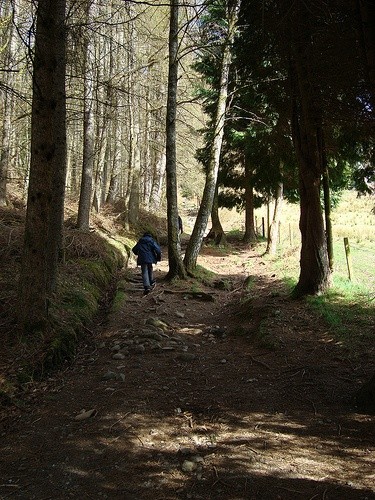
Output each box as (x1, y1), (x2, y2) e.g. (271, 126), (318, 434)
(178, 215), (184, 242)
(131, 230), (162, 296)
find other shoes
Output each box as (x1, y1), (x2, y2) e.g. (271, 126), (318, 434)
(151, 281), (155, 286)
(143, 289), (150, 295)
